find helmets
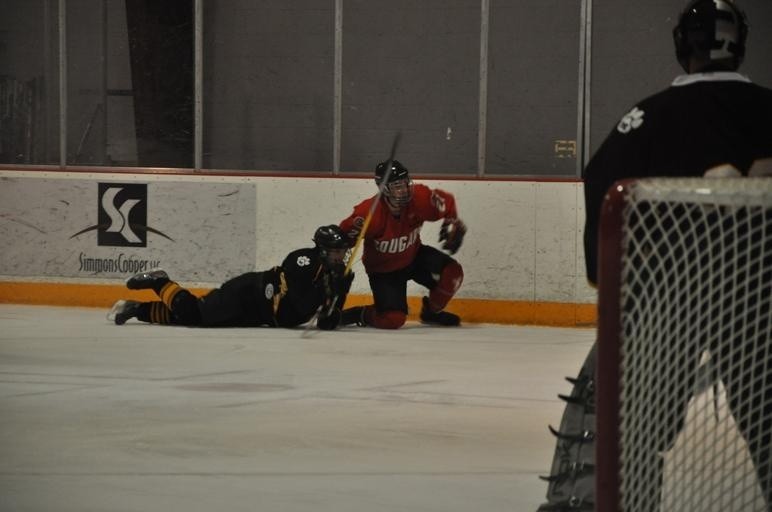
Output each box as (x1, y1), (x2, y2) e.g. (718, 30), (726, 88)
(312, 223), (355, 250)
(372, 159), (410, 186)
(668, 0), (753, 73)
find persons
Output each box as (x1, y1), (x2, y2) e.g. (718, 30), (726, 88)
(538, 2), (772, 511)
(114, 225), (351, 328)
(339, 160), (466, 326)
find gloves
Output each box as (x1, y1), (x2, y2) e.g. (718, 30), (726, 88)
(327, 267), (358, 297)
(439, 217), (469, 256)
(316, 303), (343, 331)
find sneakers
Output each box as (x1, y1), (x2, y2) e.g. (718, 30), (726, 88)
(125, 268), (170, 291)
(339, 304), (366, 328)
(419, 296), (462, 327)
(114, 297), (143, 327)
